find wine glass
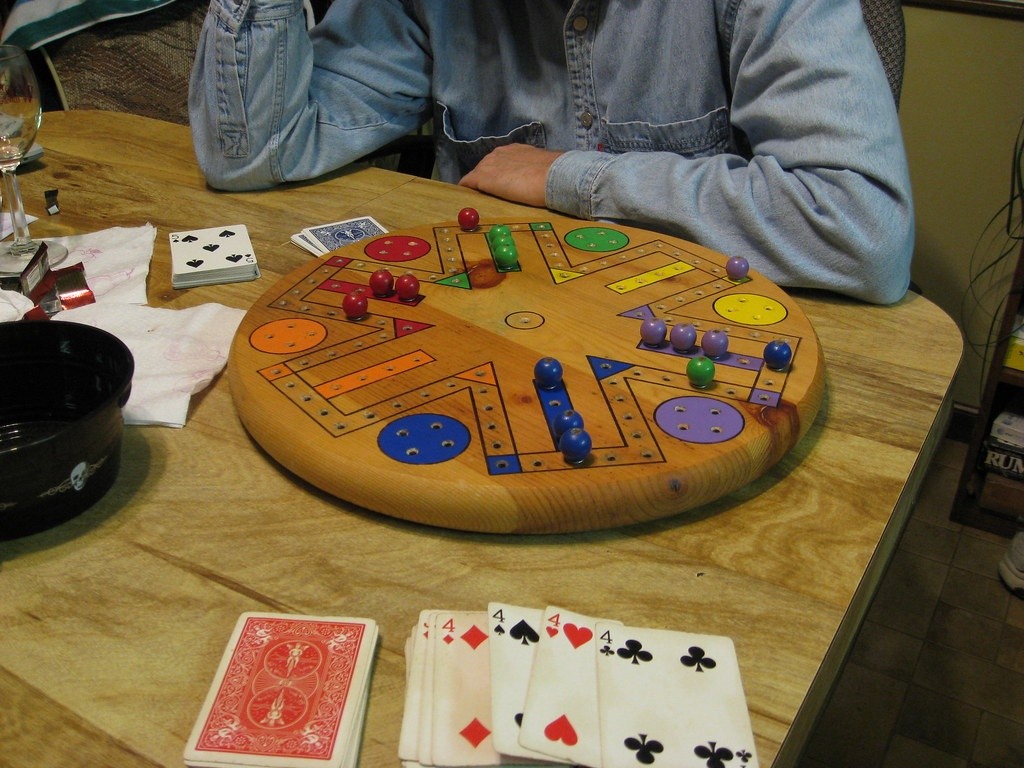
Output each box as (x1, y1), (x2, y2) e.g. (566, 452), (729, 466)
(0, 43), (68, 278)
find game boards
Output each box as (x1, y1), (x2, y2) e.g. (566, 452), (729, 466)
(228, 214), (830, 536)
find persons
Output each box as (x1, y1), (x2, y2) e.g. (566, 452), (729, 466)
(187, 0), (915, 307)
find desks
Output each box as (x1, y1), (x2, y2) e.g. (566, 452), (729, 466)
(0, 106), (965, 768)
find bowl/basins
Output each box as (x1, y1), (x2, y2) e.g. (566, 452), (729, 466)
(0, 318), (137, 542)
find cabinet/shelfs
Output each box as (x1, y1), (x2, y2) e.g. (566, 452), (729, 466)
(949, 245), (1024, 535)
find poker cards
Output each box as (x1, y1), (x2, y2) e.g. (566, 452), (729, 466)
(291, 214), (391, 258)
(181, 599), (761, 768)
(167, 223), (262, 290)
